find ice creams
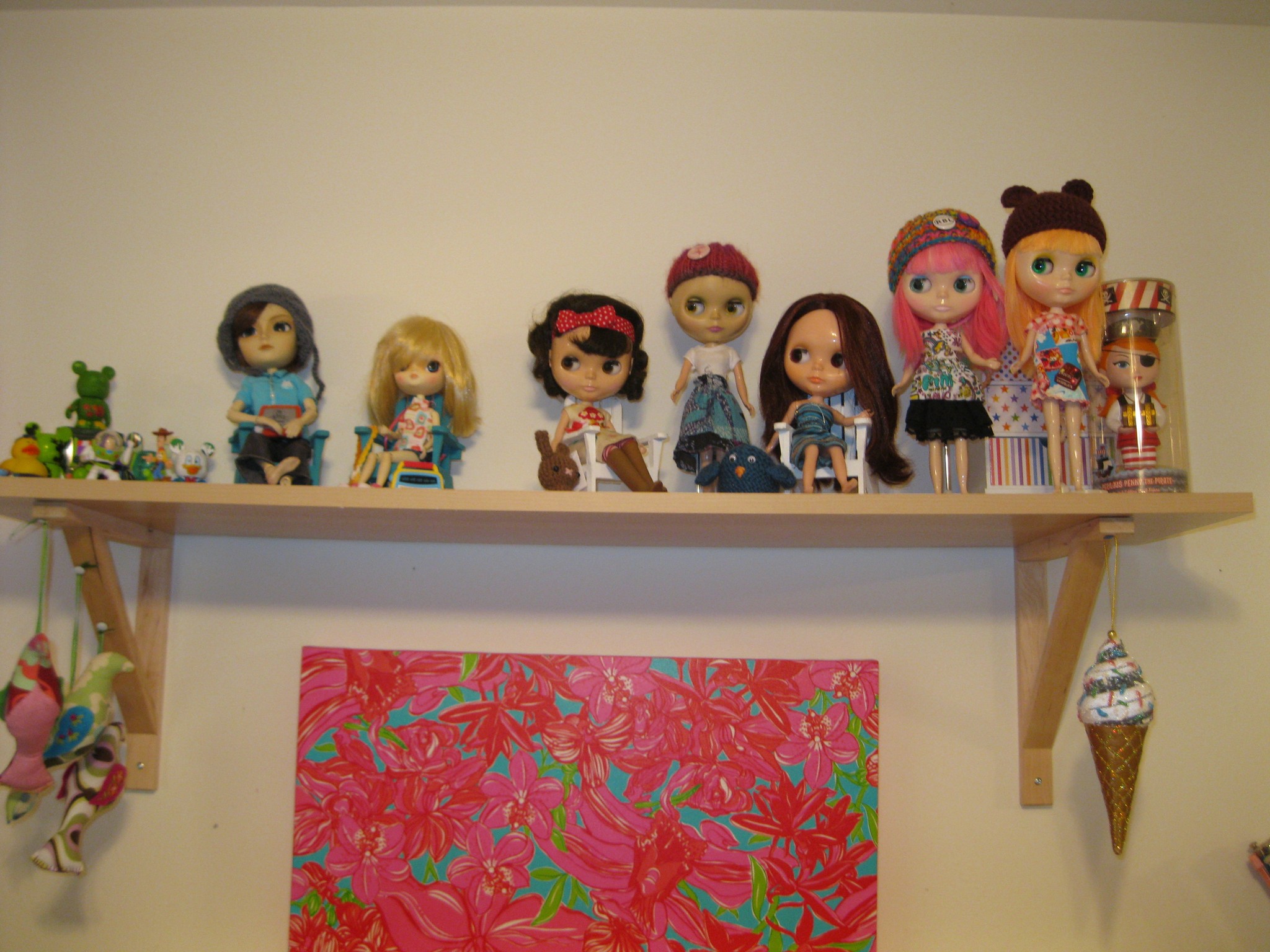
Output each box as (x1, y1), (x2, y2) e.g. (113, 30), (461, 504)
(1078, 638), (1156, 857)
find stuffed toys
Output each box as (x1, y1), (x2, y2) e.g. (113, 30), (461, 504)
(3, 650), (137, 821)
(30, 720), (130, 877)
(0, 634), (62, 795)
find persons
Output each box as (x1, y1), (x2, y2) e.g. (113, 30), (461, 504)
(662, 240), (759, 493)
(1096, 314), (1171, 475)
(883, 206), (1009, 496)
(999, 176), (1110, 493)
(347, 315), (480, 495)
(757, 291), (915, 494)
(525, 294), (671, 494)
(214, 282), (323, 488)
